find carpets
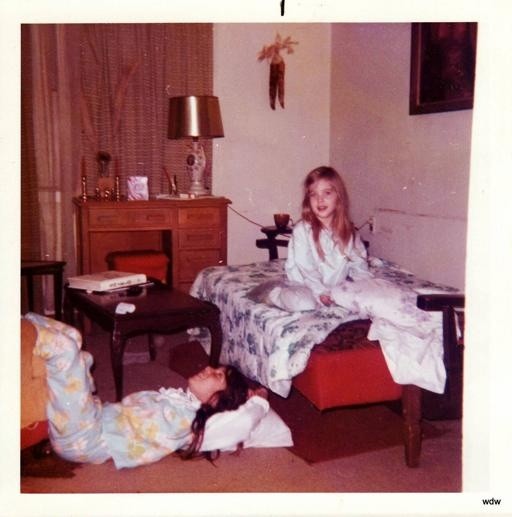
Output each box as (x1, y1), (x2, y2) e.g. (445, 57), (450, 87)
(157, 343), (444, 466)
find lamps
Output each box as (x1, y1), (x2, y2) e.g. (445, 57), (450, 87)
(168, 95), (224, 195)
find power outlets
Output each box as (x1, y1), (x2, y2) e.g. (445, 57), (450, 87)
(368, 216), (376, 233)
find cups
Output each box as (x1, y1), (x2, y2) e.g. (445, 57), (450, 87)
(273, 214), (290, 230)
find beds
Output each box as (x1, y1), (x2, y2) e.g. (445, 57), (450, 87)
(185, 239), (465, 469)
(21, 354), (52, 458)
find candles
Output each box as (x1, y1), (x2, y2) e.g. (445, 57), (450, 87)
(115, 160), (119, 175)
(81, 156), (86, 175)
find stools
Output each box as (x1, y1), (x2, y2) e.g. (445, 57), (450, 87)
(22, 261), (67, 320)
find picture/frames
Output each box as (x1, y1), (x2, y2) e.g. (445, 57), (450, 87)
(409, 23), (477, 114)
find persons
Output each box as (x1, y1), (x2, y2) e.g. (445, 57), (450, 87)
(285, 164), (367, 311)
(22, 309), (270, 469)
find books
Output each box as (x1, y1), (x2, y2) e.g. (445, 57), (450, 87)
(67, 270), (146, 291)
(128, 175), (149, 200)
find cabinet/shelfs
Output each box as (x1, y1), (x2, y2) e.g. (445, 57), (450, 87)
(72, 195), (232, 294)
